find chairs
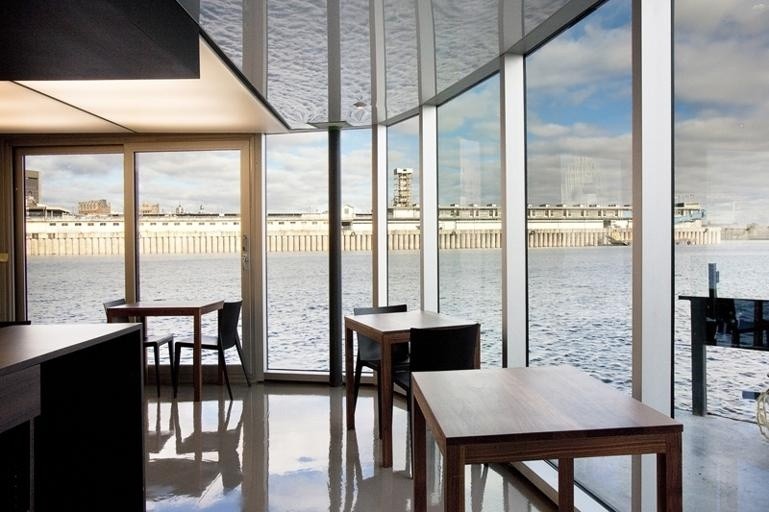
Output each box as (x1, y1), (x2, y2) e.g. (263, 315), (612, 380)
(353, 303), (482, 478)
(103, 297), (252, 400)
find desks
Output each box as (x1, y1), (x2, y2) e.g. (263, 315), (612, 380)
(0, 323), (148, 511)
(411, 363), (686, 511)
